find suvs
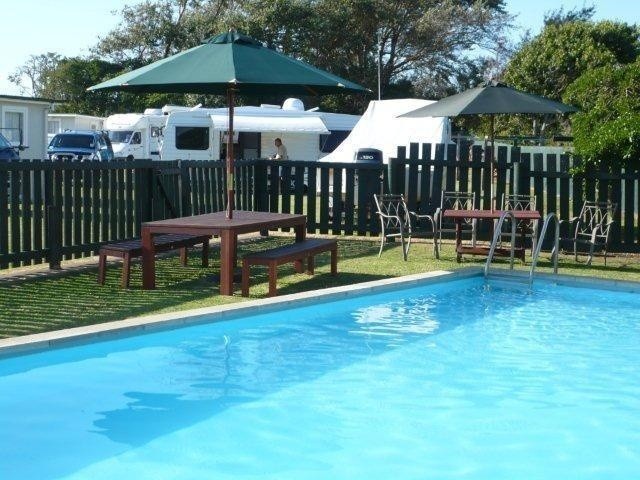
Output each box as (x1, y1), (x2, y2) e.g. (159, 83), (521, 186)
(47, 129), (113, 162)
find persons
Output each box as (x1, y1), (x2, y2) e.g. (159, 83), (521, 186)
(267, 137), (290, 195)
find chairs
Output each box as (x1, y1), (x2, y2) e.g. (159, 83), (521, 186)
(373, 193), (439, 261)
(499, 193), (538, 248)
(551, 201), (619, 266)
(433, 191), (478, 258)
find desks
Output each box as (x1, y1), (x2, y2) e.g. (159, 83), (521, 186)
(443, 210), (541, 263)
(140, 210), (308, 296)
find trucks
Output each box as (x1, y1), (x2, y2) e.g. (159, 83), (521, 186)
(102, 111), (220, 161)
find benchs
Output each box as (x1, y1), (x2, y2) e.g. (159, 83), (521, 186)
(98, 234), (212, 288)
(241, 239), (337, 297)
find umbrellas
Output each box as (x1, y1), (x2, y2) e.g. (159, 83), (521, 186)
(394, 79), (578, 244)
(90, 337), (341, 470)
(84, 25), (376, 266)
